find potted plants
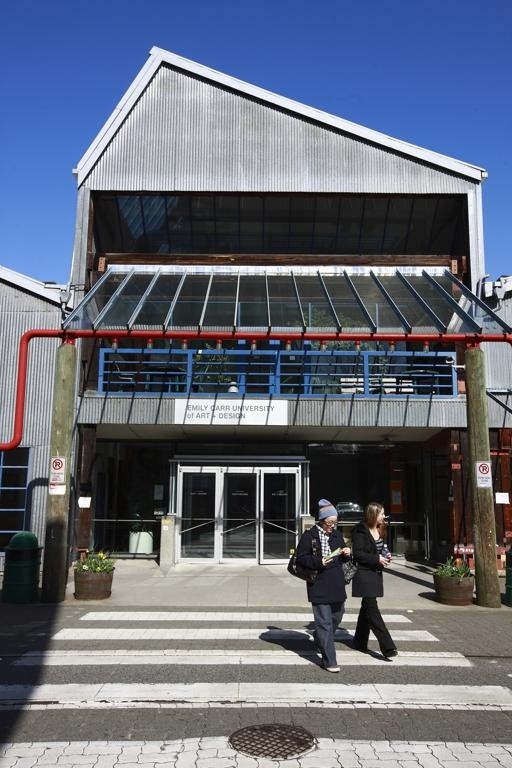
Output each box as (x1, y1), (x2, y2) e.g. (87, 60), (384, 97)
(432, 557), (475, 606)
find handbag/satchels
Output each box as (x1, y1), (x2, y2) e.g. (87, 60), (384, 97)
(287, 530), (319, 584)
(342, 555), (359, 585)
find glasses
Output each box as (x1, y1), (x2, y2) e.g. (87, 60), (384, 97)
(324, 520), (339, 526)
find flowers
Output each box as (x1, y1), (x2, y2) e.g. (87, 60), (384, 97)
(74, 548), (115, 574)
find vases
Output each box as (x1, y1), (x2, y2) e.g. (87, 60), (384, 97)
(73, 569), (114, 600)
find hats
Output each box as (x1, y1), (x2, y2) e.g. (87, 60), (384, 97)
(318, 498), (339, 520)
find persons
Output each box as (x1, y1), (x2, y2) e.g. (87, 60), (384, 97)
(295, 514), (352, 673)
(349, 502), (399, 659)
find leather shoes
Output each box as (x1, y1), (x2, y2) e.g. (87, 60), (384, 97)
(313, 631), (321, 653)
(325, 667), (340, 673)
(384, 649), (398, 659)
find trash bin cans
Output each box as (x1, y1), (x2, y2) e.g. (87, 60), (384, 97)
(4, 532), (44, 604)
